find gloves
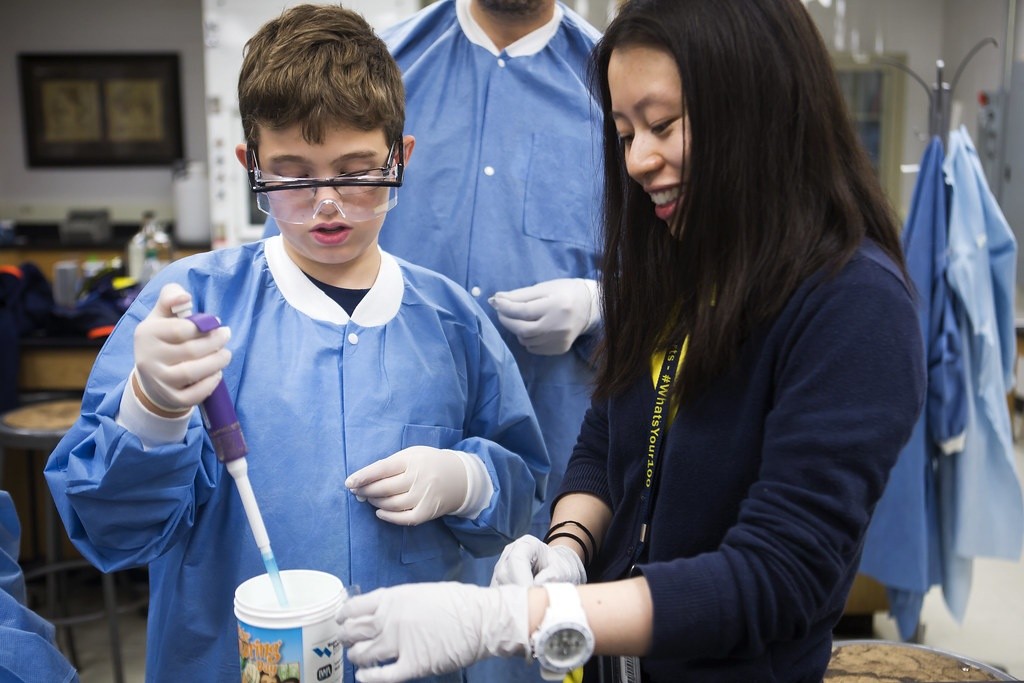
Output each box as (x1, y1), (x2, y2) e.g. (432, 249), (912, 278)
(345, 445), (494, 525)
(489, 534), (587, 586)
(489, 279), (604, 357)
(338, 580), (530, 683)
(133, 283), (232, 412)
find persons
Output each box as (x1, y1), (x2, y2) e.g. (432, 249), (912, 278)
(43, 5), (553, 683)
(262, 0), (606, 542)
(335, 0), (924, 683)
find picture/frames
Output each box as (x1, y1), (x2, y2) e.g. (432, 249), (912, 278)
(15, 51), (186, 173)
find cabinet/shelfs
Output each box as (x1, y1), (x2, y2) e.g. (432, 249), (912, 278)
(827, 48), (908, 229)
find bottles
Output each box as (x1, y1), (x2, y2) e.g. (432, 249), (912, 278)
(172, 160), (208, 245)
(127, 211), (172, 286)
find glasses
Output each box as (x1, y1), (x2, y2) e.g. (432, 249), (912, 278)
(252, 138), (397, 199)
(247, 138), (404, 223)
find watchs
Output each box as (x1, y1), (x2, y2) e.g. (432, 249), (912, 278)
(531, 582), (594, 681)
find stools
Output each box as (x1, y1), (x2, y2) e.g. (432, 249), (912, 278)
(0, 398), (151, 682)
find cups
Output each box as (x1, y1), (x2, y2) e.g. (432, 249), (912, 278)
(52, 261), (80, 303)
(234, 570), (346, 683)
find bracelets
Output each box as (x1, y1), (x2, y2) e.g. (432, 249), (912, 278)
(543, 521), (596, 569)
